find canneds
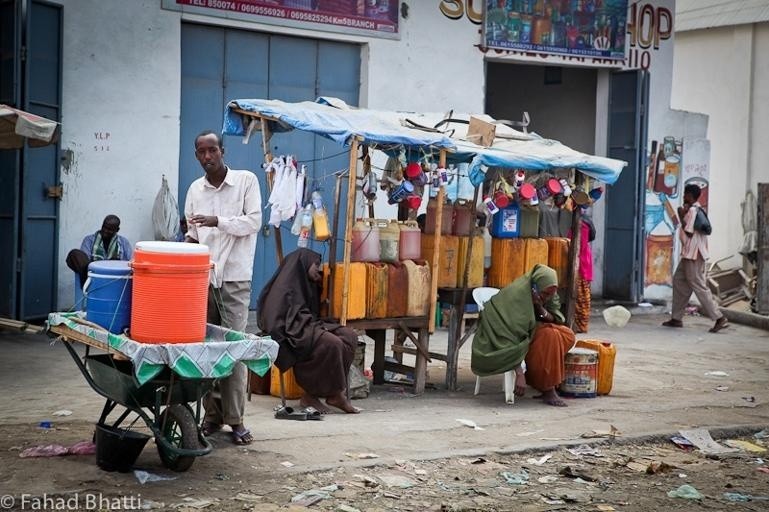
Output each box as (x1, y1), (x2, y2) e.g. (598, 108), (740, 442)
(684, 178), (709, 216)
(664, 156), (680, 188)
(506, 12), (523, 43)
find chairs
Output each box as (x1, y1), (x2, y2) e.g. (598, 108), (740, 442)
(471, 286), (528, 404)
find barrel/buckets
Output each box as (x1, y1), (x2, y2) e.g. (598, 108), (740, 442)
(454, 198), (474, 236)
(129, 242), (215, 344)
(562, 350), (599, 396)
(426, 197), (453, 234)
(95, 415), (151, 471)
(576, 339), (616, 393)
(83, 261), (132, 334)
(330, 219), (430, 320)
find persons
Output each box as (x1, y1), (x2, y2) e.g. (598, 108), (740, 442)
(564, 198), (593, 333)
(469, 263), (576, 407)
(180, 131), (263, 445)
(662, 184), (729, 332)
(257, 247), (360, 415)
(65, 214), (133, 311)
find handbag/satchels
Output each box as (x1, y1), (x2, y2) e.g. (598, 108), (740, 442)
(686, 204), (712, 235)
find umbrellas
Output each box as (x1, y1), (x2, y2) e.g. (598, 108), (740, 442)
(0, 103), (63, 149)
(737, 188), (758, 295)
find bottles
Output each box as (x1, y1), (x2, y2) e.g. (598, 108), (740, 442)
(369, 172), (377, 197)
(290, 187), (326, 250)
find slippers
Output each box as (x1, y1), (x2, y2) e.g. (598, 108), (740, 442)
(663, 321), (683, 327)
(273, 403), (325, 421)
(233, 429), (250, 445)
(709, 320), (729, 332)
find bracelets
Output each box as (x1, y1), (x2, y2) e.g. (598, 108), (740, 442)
(538, 309), (547, 318)
